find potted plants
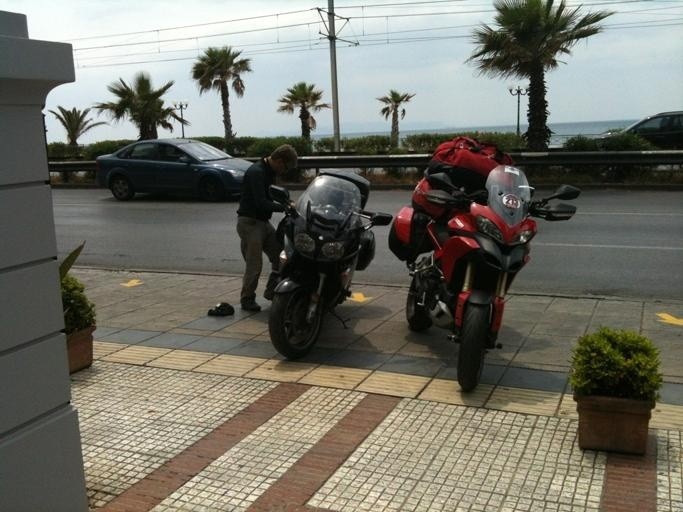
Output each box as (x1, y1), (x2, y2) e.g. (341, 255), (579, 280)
(563, 320), (664, 459)
(59, 272), (98, 375)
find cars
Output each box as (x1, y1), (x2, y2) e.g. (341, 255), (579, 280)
(95, 136), (253, 203)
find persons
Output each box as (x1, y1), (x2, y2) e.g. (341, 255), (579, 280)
(237, 145), (298, 313)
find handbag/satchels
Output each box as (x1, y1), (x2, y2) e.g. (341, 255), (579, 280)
(410, 135), (520, 215)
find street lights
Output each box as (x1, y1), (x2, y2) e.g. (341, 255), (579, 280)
(171, 100), (191, 142)
(503, 81), (530, 140)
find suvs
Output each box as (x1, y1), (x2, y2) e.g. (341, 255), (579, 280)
(592, 110), (683, 165)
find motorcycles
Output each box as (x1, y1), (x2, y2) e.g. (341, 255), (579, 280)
(262, 164), (395, 361)
(384, 136), (581, 400)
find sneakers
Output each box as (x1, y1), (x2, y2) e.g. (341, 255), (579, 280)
(239, 298), (261, 313)
